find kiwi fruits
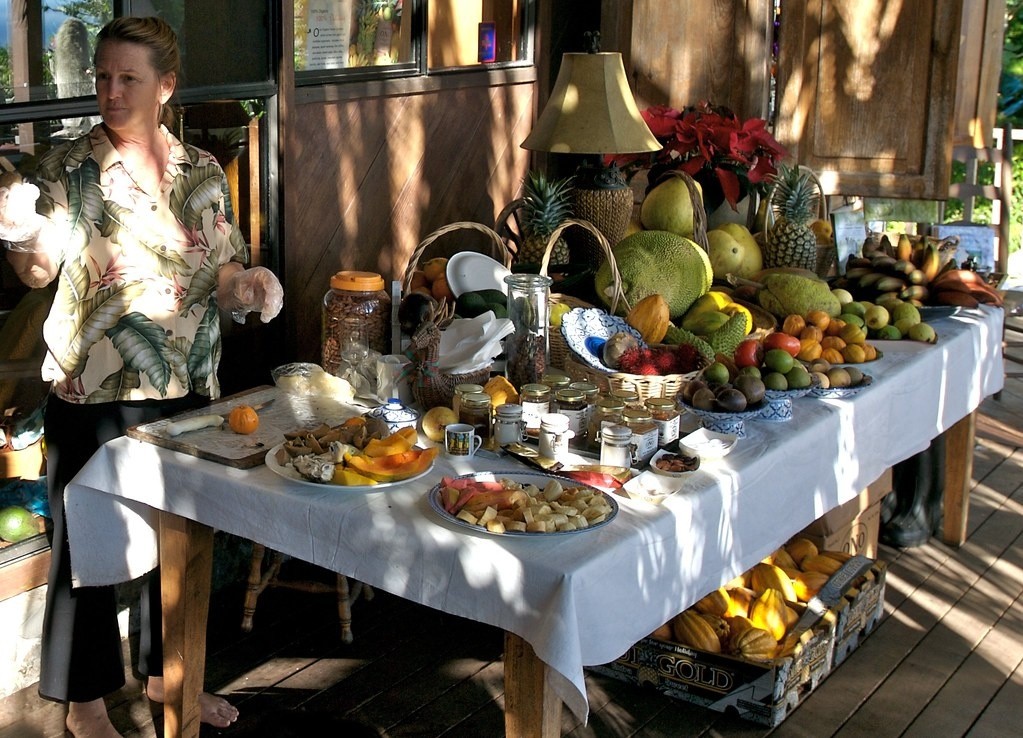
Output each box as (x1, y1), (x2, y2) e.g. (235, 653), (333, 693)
(684, 381), (715, 411)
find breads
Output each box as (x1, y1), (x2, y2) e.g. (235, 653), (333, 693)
(439, 477), (613, 533)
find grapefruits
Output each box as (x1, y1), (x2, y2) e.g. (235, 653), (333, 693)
(625, 176), (763, 280)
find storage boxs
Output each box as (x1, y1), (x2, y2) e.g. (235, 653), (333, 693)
(583, 560), (887, 726)
(798, 499), (883, 558)
(800, 466), (894, 535)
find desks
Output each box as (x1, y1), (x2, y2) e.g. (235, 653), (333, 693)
(108, 306), (1005, 738)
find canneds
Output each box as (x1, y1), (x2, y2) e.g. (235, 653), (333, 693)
(453, 375), (679, 467)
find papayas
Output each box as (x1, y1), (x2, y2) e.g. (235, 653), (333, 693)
(333, 426), (439, 486)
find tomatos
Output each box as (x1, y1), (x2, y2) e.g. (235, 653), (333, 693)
(734, 340), (763, 368)
(766, 334), (800, 356)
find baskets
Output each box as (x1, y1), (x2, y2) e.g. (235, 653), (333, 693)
(401, 170), (714, 413)
(754, 165), (836, 278)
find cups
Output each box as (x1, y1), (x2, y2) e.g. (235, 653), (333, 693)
(445, 424), (482, 461)
(368, 398), (419, 435)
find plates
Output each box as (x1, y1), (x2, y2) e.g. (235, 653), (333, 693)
(265, 442), (435, 491)
(428, 471), (619, 536)
(917, 305), (960, 321)
(675, 389), (769, 440)
(809, 372), (875, 399)
(749, 386), (816, 422)
(830, 346), (884, 365)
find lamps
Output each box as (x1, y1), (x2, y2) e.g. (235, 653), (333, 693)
(519, 31), (664, 247)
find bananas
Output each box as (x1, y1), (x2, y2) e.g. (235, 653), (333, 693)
(830, 234), (1003, 307)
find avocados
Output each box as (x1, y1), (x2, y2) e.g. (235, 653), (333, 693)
(451, 289), (507, 319)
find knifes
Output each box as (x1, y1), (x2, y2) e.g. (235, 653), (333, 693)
(166, 399), (276, 436)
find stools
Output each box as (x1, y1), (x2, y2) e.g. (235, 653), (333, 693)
(244, 542), (373, 644)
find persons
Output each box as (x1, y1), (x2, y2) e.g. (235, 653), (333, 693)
(0, 18), (283, 738)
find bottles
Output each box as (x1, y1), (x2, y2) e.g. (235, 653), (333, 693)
(596, 426), (632, 470)
(539, 413), (575, 459)
(492, 403), (527, 445)
(320, 271), (391, 377)
(503, 273), (554, 395)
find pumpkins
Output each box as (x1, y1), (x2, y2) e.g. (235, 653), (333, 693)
(345, 418), (365, 427)
(652, 538), (874, 661)
(229, 405), (258, 435)
(784, 311), (876, 363)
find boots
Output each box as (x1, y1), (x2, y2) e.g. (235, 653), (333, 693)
(878, 477), (945, 546)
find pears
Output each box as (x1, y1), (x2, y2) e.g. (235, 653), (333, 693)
(422, 407), (457, 441)
(812, 219), (832, 240)
(832, 289), (935, 343)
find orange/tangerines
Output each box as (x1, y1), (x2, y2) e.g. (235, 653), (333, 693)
(411, 259), (450, 299)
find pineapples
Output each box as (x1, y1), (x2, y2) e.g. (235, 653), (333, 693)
(517, 170), (577, 264)
(765, 163), (817, 272)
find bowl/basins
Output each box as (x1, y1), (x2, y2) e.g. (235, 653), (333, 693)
(679, 427), (738, 463)
(649, 448), (700, 480)
(560, 307), (648, 372)
(622, 470), (684, 506)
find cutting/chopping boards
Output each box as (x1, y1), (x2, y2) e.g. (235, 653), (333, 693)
(126, 385), (371, 470)
